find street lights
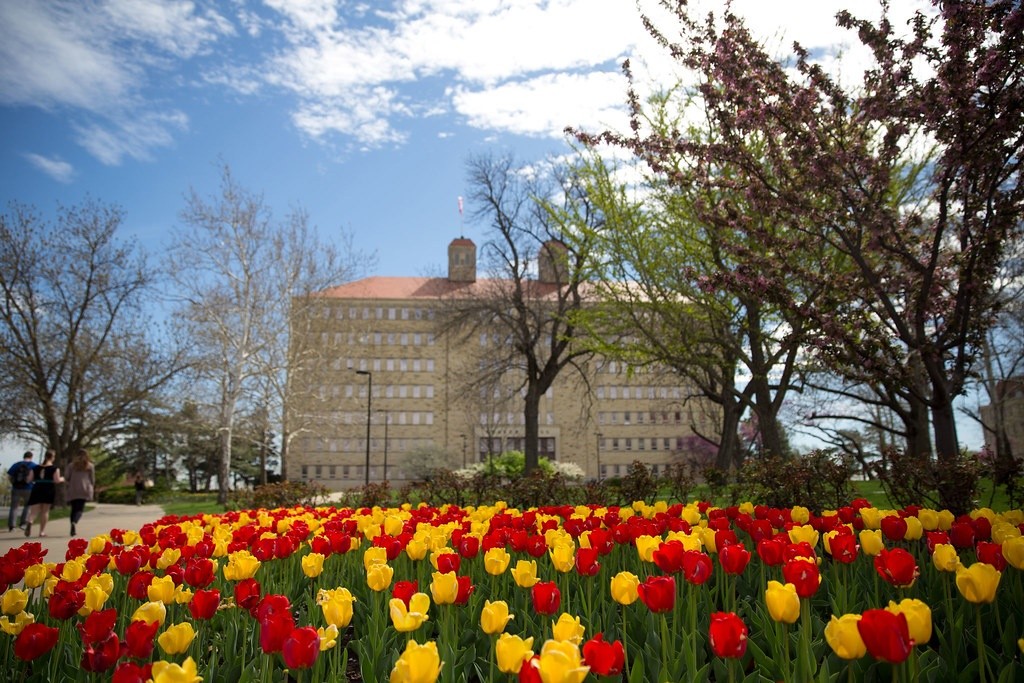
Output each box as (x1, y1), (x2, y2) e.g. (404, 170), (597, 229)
(460, 433), (468, 469)
(593, 431), (603, 481)
(376, 409), (388, 480)
(356, 370), (371, 486)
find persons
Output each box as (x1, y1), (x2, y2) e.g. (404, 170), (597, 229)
(23, 451), (65, 537)
(135, 474), (144, 506)
(7, 452), (38, 533)
(61, 448), (96, 536)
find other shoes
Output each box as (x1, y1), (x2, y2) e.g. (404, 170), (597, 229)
(8, 526), (16, 533)
(19, 525), (25, 530)
(70, 523), (76, 536)
(39, 534), (48, 537)
(25, 522), (32, 537)
